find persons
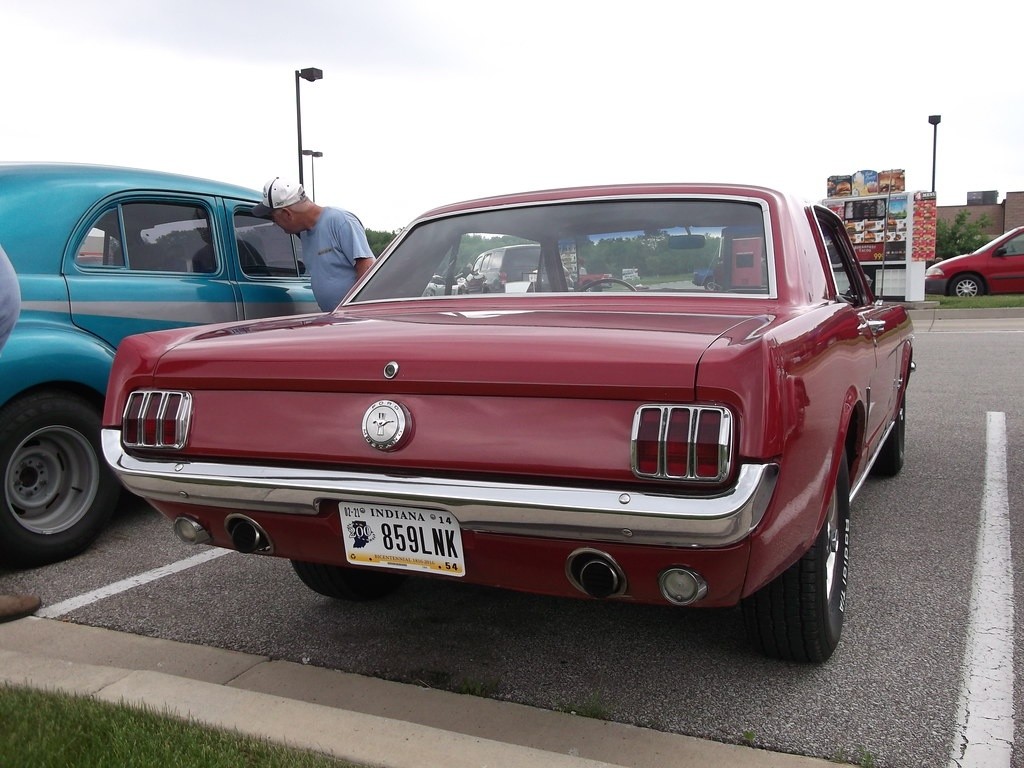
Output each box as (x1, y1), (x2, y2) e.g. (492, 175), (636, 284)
(0, 245), (41, 624)
(251, 177), (377, 313)
(193, 208), (288, 325)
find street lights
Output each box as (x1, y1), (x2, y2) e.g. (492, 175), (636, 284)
(927, 114), (941, 193)
(294, 66), (325, 186)
(302, 150), (325, 204)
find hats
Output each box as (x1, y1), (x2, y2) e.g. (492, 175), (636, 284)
(192, 207), (209, 231)
(251, 177), (304, 217)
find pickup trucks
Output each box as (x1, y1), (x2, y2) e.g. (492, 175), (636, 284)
(573, 273), (615, 292)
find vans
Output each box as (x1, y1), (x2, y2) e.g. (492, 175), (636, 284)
(465, 243), (571, 293)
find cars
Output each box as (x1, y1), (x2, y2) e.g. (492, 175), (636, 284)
(421, 272), (467, 296)
(1, 157), (325, 571)
(691, 267), (717, 291)
(99, 183), (917, 664)
(926, 224), (1024, 298)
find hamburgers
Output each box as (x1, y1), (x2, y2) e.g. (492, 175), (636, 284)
(846, 218), (897, 242)
(912, 204), (934, 252)
(836, 181), (851, 193)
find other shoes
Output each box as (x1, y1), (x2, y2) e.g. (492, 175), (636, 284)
(0, 594), (41, 623)
